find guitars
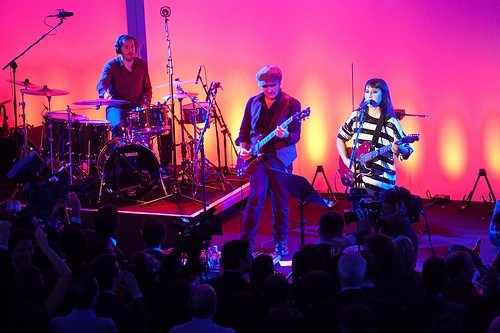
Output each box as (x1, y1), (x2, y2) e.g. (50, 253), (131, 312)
(339, 134), (419, 187)
(236, 107), (311, 180)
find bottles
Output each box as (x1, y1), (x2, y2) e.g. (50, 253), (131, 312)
(471, 235), (483, 256)
(207, 247), (214, 267)
(212, 246), (220, 264)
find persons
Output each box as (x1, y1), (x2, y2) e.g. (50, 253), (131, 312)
(0, 165), (500, 333)
(235, 66), (303, 256)
(338, 78), (414, 238)
(96, 34), (153, 140)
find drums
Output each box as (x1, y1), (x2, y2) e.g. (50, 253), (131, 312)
(44, 111), (89, 163)
(182, 102), (216, 124)
(96, 137), (162, 202)
(73, 119), (112, 163)
(127, 102), (171, 140)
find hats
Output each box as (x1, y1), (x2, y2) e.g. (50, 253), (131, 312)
(256, 65), (282, 86)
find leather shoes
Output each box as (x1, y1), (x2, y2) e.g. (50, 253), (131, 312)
(275, 242), (288, 255)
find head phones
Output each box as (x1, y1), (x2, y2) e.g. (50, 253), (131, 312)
(393, 186), (406, 215)
(115, 35), (137, 54)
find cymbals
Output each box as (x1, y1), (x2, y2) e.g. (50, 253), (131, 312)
(72, 99), (131, 106)
(154, 81), (197, 88)
(6, 79), (41, 88)
(20, 88), (71, 96)
(176, 93), (199, 98)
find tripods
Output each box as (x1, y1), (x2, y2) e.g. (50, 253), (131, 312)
(2, 17), (90, 187)
(139, 16), (246, 208)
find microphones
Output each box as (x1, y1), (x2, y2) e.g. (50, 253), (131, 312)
(162, 9), (169, 17)
(359, 99), (371, 109)
(48, 12), (73, 18)
(141, 173), (150, 181)
(195, 66), (201, 84)
